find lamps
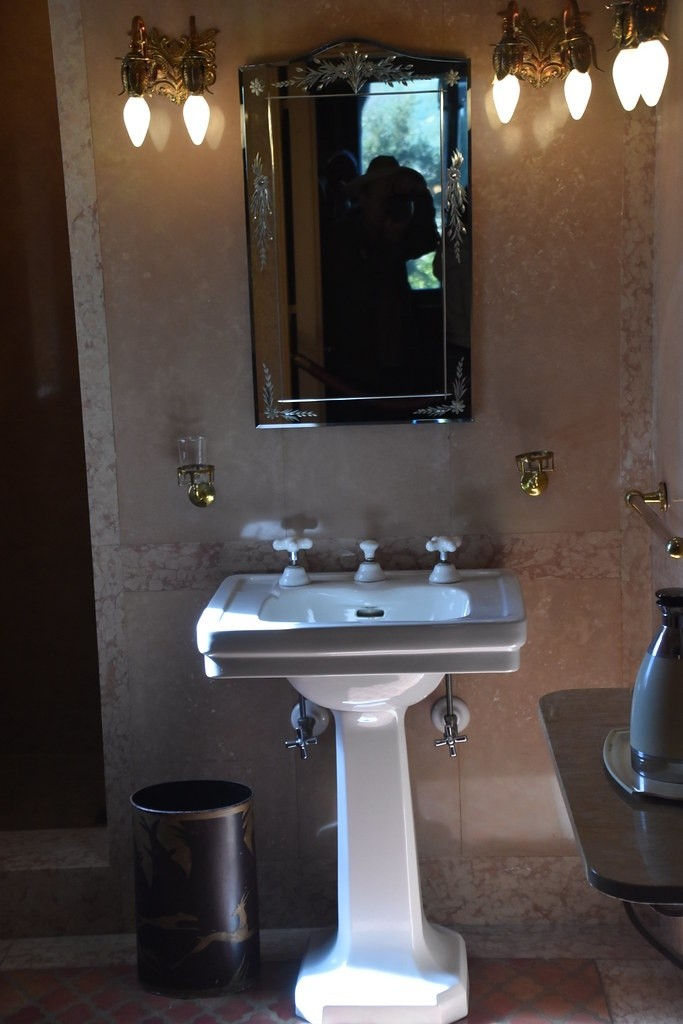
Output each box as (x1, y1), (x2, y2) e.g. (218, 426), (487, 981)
(608, 0), (671, 113)
(117, 14), (220, 148)
(491, 0), (604, 124)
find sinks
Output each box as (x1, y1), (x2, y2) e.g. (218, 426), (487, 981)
(196, 564), (529, 678)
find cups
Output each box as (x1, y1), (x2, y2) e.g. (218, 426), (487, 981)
(177, 436), (211, 486)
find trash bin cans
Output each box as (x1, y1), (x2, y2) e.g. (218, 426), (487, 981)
(128, 779), (262, 1000)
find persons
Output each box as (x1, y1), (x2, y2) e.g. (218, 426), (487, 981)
(319, 155), (440, 384)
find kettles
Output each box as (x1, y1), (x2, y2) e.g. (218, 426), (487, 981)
(631, 587), (682, 784)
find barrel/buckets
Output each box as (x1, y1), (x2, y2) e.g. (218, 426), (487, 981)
(127, 776), (265, 998)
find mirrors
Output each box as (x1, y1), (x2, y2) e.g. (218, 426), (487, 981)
(240, 40), (473, 427)
